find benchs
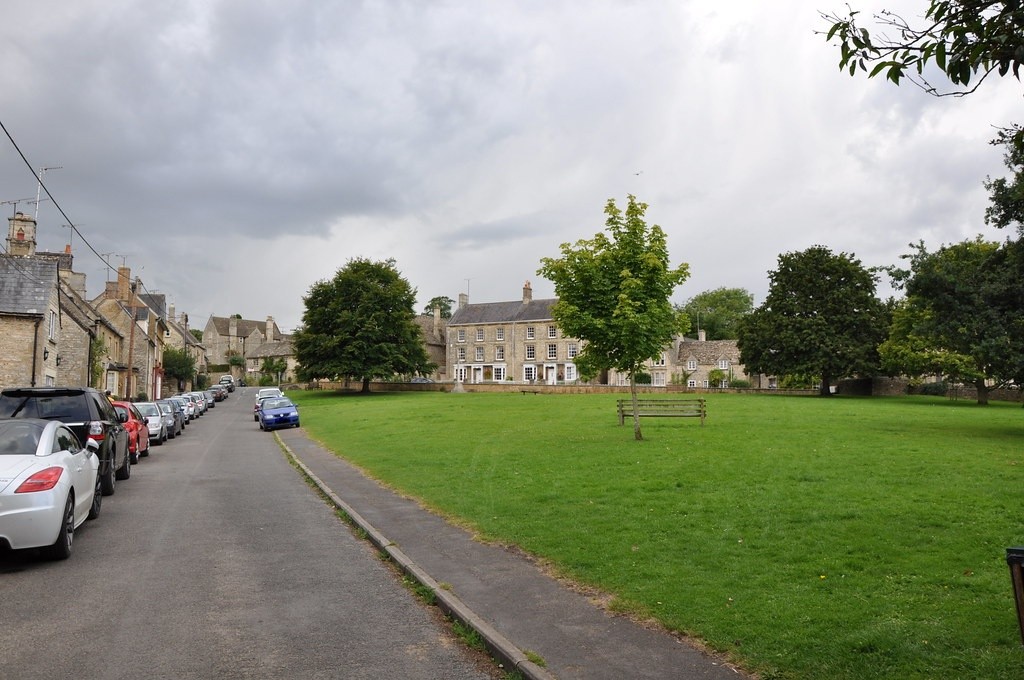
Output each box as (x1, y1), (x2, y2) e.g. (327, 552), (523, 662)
(616, 399), (707, 428)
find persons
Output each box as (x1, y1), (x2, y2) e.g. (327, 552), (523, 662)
(106, 390), (114, 401)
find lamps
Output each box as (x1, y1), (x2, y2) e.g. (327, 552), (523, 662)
(56, 355), (61, 366)
(44, 347), (49, 361)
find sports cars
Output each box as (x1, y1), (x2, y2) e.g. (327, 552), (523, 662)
(0, 416), (101, 569)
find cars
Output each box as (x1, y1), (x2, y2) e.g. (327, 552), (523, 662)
(130, 402), (169, 446)
(152, 373), (238, 440)
(252, 388), (287, 420)
(106, 401), (151, 463)
(254, 398), (300, 431)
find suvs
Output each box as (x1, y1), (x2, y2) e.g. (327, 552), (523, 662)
(0, 386), (132, 496)
